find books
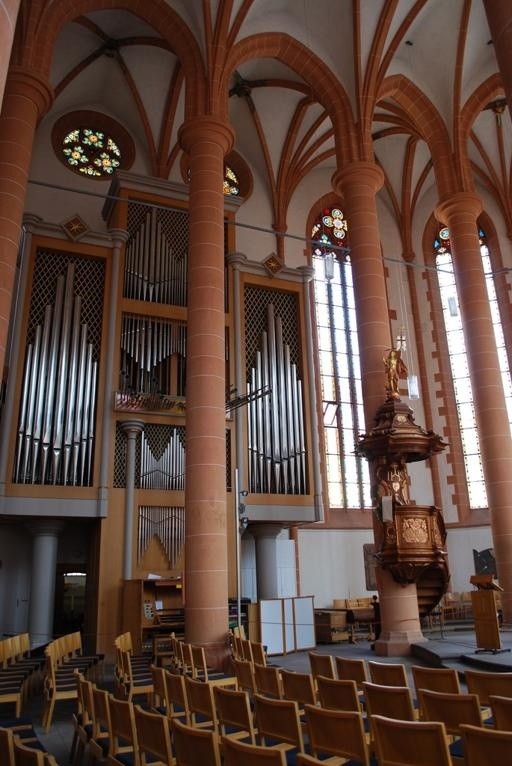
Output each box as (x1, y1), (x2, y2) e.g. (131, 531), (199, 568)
(228, 606), (247, 633)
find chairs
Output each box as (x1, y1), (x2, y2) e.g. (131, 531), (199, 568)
(334, 590), (473, 643)
(0, 626), (512, 766)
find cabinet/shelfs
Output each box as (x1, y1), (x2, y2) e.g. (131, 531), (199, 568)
(121, 580), (184, 655)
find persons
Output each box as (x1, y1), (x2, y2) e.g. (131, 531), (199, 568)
(369, 595), (382, 641)
